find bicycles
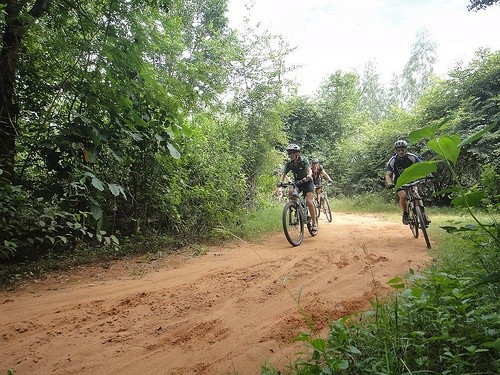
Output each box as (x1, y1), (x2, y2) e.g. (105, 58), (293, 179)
(276, 181), (319, 247)
(392, 177), (433, 249)
(311, 182), (333, 222)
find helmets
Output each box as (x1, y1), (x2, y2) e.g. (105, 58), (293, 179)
(311, 158), (319, 163)
(285, 143), (300, 153)
(394, 140), (407, 148)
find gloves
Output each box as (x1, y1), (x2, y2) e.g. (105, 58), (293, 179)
(296, 180), (303, 185)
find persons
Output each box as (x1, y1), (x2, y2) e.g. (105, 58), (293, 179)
(385, 140), (434, 225)
(278, 144), (318, 232)
(311, 158), (333, 209)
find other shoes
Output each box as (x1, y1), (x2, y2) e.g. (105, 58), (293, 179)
(402, 215), (409, 225)
(423, 216), (431, 222)
(312, 223), (318, 230)
